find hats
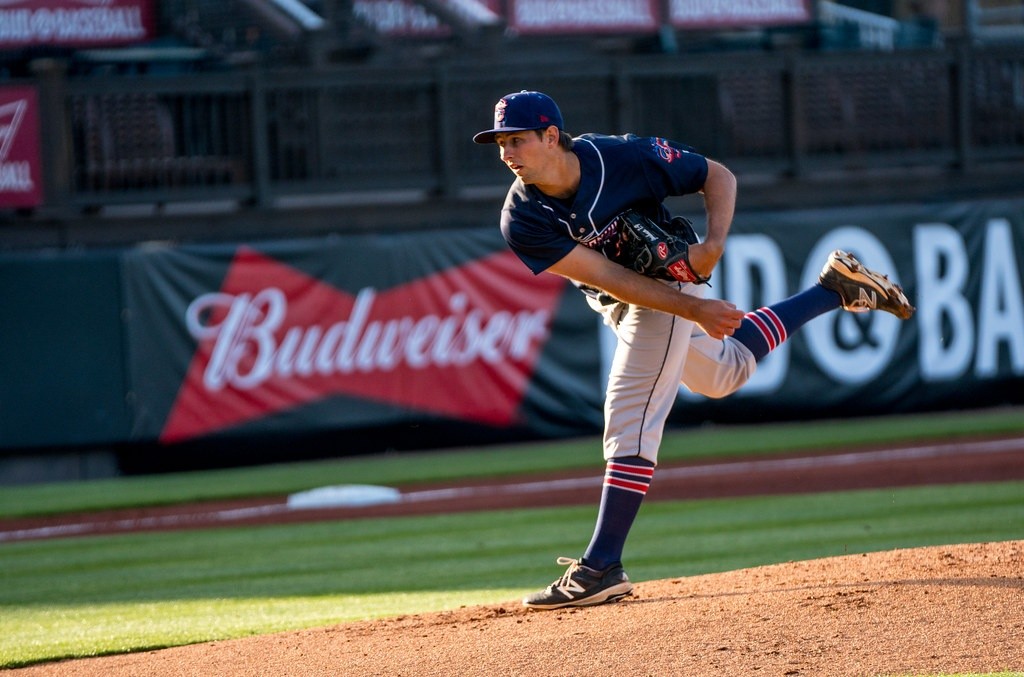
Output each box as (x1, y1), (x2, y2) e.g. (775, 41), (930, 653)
(472, 89), (564, 143)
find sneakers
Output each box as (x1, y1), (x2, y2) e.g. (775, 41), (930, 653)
(818, 249), (915, 320)
(523, 557), (634, 609)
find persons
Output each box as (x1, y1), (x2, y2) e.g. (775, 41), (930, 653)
(472, 87), (920, 611)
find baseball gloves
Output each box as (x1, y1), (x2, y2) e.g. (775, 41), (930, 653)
(610, 210), (713, 288)
